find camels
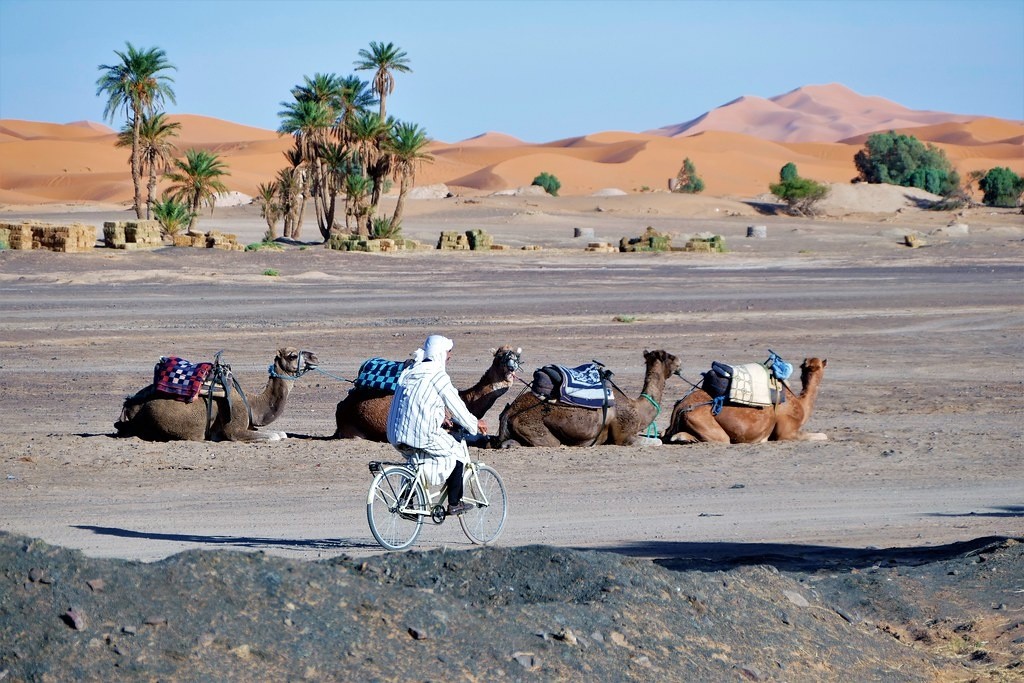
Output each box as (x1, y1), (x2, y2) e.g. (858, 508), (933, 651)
(335, 344), (522, 439)
(119, 347), (320, 442)
(660, 356), (827, 445)
(493, 349), (683, 450)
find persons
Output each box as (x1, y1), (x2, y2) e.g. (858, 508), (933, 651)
(388, 334), (489, 517)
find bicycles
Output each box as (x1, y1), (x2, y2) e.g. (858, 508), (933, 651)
(365, 422), (509, 550)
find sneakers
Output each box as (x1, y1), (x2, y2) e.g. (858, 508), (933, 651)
(448, 501), (472, 515)
(399, 502), (417, 521)
